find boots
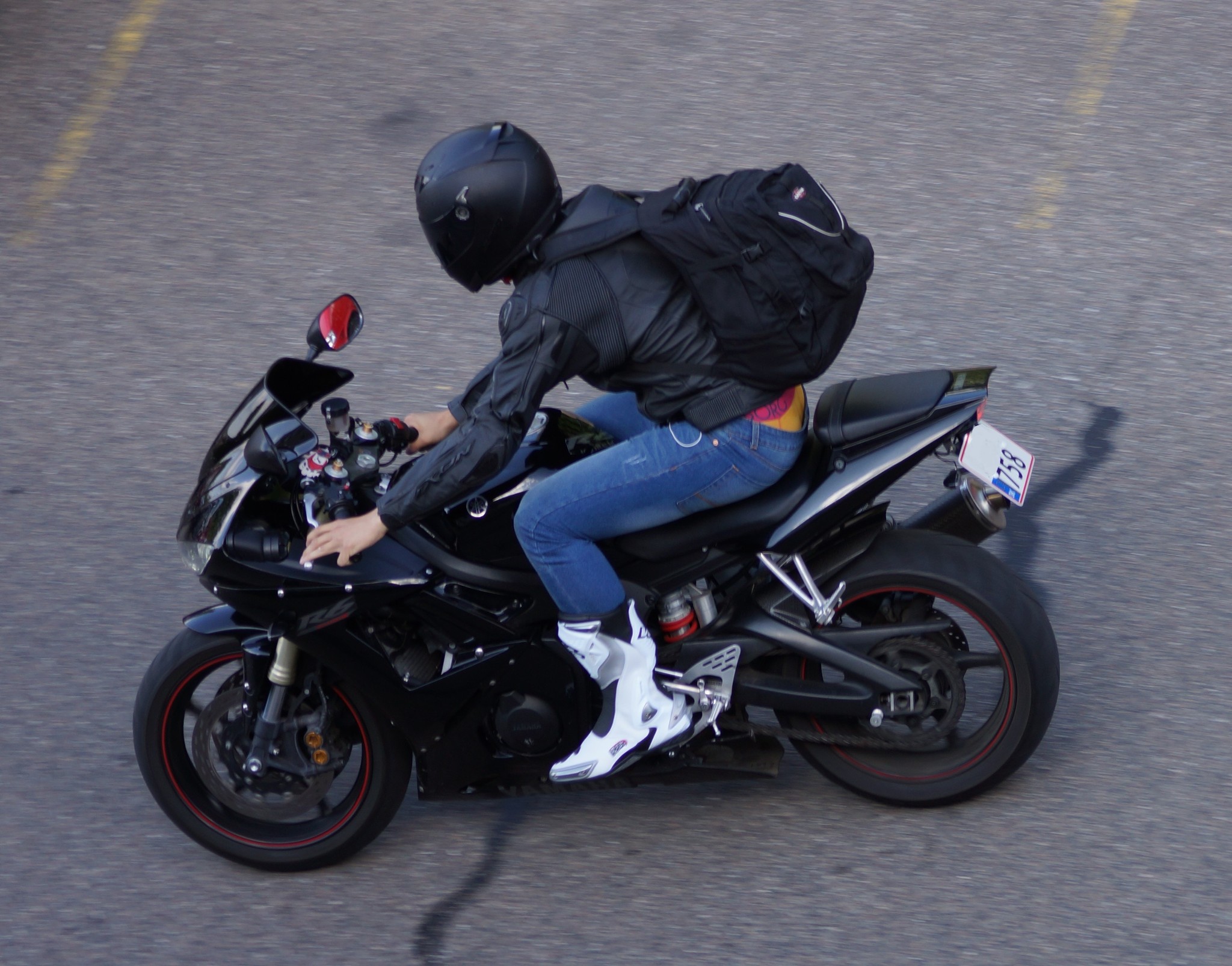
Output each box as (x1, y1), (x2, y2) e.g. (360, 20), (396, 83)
(548, 600), (694, 784)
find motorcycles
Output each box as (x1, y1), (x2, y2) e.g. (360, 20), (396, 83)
(132, 294), (1061, 874)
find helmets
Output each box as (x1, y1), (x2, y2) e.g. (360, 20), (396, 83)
(416, 122), (563, 293)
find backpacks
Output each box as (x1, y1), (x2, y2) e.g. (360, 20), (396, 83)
(545, 161), (873, 391)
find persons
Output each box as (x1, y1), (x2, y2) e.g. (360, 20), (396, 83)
(299, 119), (812, 782)
